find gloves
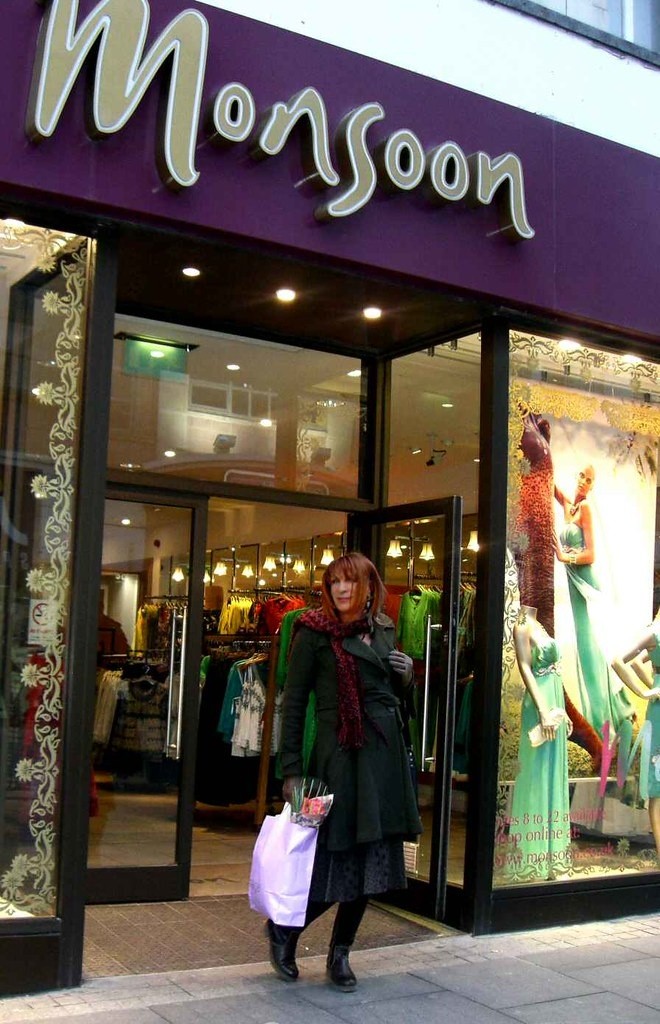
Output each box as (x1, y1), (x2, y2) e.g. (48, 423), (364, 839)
(388, 649), (414, 688)
(282, 776), (301, 806)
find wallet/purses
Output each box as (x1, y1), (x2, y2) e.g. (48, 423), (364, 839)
(528, 707), (567, 748)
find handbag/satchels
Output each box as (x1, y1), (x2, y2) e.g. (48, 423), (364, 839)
(248, 801), (320, 928)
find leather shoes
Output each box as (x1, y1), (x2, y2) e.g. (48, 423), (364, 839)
(263, 917), (300, 983)
(325, 945), (359, 993)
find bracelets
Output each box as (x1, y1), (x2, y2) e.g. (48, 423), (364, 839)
(569, 554), (576, 566)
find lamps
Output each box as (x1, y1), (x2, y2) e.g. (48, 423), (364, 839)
(171, 560), (210, 584)
(321, 533), (346, 567)
(386, 524), (435, 584)
(212, 433), (237, 453)
(309, 447), (332, 464)
(263, 543), (305, 587)
(213, 549), (254, 589)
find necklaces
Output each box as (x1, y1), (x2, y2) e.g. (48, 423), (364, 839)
(570, 497), (586, 515)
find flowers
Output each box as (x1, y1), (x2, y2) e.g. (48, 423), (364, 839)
(301, 795), (327, 824)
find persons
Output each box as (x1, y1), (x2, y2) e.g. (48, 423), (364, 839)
(611, 608), (660, 865)
(97, 587), (128, 656)
(264, 552), (418, 989)
(512, 606), (572, 882)
(536, 418), (634, 741)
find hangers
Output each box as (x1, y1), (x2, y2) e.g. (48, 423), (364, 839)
(104, 650), (169, 685)
(140, 594), (187, 611)
(228, 588), (323, 612)
(460, 571), (476, 591)
(408, 574), (441, 600)
(209, 641), (272, 670)
